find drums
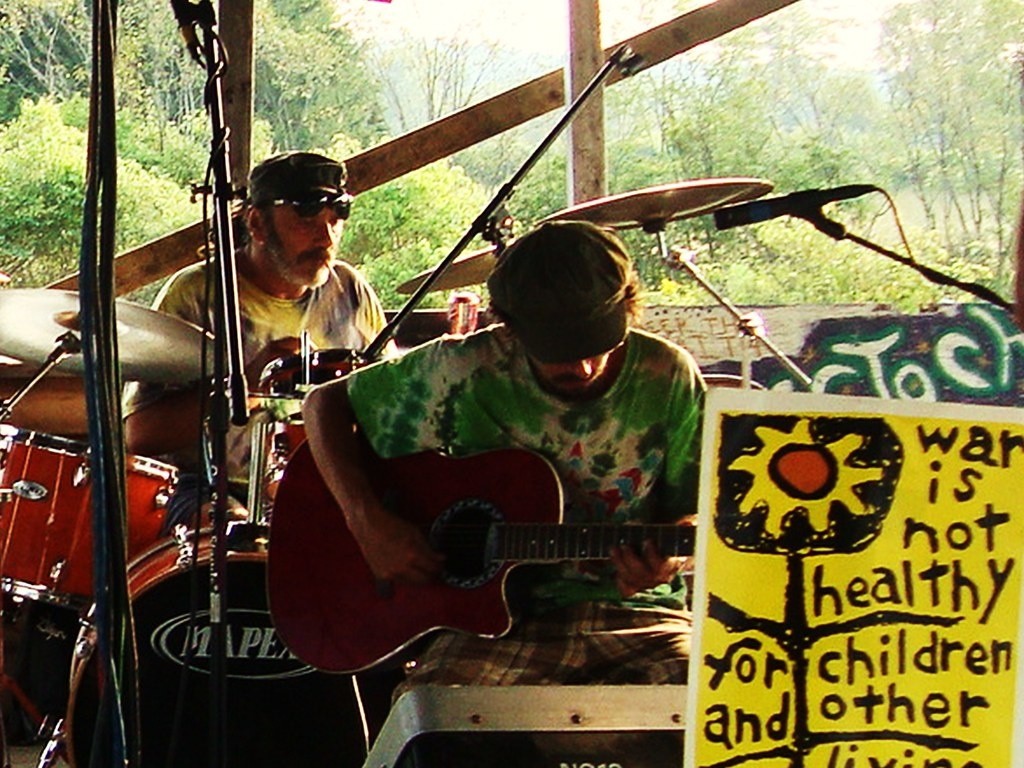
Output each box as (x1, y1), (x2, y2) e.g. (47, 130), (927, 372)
(61, 527), (396, 768)
(0, 425), (185, 638)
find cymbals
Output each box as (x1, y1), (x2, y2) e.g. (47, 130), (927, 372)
(531, 176), (775, 226)
(396, 232), (540, 292)
(0, 284), (231, 388)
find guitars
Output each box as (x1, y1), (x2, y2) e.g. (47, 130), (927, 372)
(265, 425), (698, 674)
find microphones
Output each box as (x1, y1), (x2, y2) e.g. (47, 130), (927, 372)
(171, 0), (201, 61)
(715, 185), (875, 231)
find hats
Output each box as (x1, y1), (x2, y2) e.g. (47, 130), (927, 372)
(486, 221), (633, 364)
(250, 151), (348, 203)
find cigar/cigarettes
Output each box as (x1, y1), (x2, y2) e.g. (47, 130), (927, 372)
(321, 258), (345, 294)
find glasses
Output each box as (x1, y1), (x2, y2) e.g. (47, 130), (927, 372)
(268, 195), (351, 221)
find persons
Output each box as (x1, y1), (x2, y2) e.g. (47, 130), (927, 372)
(120, 151), (396, 529)
(302, 217), (710, 704)
